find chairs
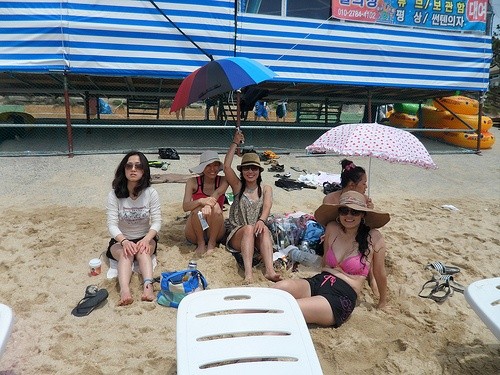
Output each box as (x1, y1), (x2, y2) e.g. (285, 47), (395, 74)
(175, 287), (324, 375)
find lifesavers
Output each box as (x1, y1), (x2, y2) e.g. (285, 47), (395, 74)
(388, 96), (496, 149)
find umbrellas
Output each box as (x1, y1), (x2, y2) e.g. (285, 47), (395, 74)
(305, 122), (439, 205)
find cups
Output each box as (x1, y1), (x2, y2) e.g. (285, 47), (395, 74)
(89, 259), (101, 277)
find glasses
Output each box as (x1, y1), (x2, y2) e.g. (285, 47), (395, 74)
(243, 166), (259, 171)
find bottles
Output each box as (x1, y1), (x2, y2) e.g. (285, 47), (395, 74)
(288, 249), (322, 270)
(188, 261), (198, 272)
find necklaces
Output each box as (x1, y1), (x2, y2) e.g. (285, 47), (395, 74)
(246, 184), (258, 193)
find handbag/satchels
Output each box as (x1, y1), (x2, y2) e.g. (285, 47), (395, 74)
(157, 269), (207, 308)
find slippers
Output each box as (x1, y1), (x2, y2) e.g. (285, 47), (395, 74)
(437, 274), (466, 294)
(418, 275), (454, 303)
(70, 288), (109, 317)
(424, 262), (461, 274)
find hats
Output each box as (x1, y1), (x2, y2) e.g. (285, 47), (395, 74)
(338, 207), (361, 216)
(314, 191), (391, 229)
(189, 150), (224, 175)
(237, 153), (264, 172)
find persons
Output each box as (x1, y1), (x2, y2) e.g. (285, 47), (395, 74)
(183, 151), (229, 260)
(387, 105), (396, 118)
(322, 159), (376, 210)
(253, 100), (269, 121)
(275, 101), (288, 123)
(202, 95), (218, 120)
(169, 97), (185, 120)
(261, 191), (388, 362)
(224, 130), (283, 285)
(169, 55), (280, 144)
(105, 152), (162, 305)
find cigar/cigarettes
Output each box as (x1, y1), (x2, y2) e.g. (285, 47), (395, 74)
(255, 232), (258, 237)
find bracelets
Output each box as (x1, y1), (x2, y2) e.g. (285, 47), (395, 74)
(233, 142), (239, 147)
(257, 219), (265, 224)
(121, 239), (129, 246)
